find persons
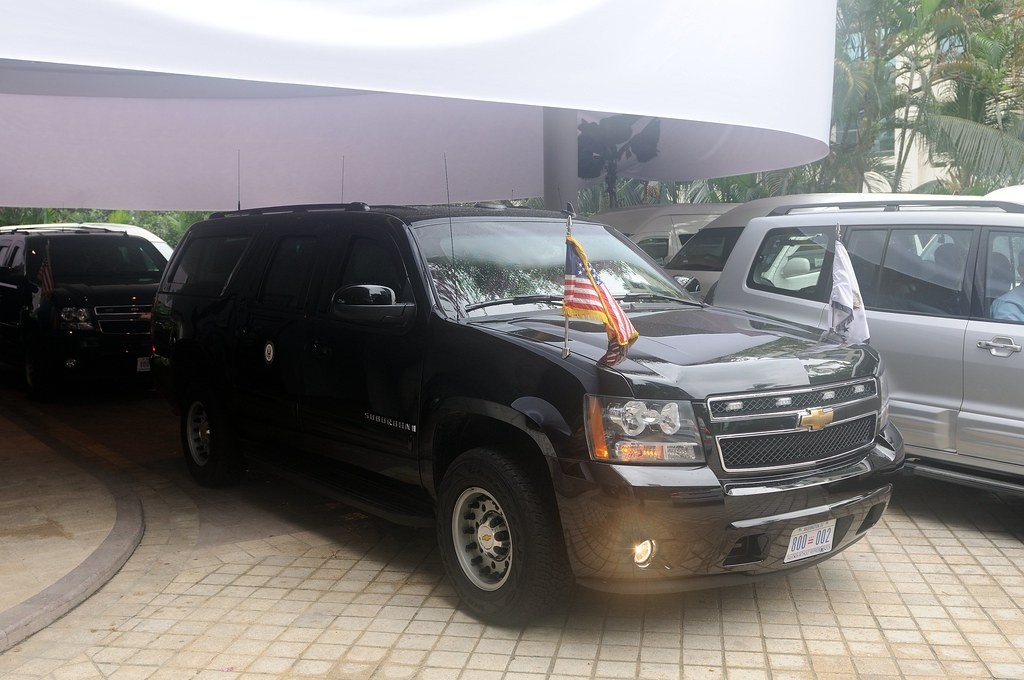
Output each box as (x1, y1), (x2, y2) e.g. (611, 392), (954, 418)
(990, 249), (1024, 322)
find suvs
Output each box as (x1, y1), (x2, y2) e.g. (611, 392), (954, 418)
(1, 223), (176, 414)
(578, 191), (740, 263)
(153, 199), (907, 635)
(664, 192), (1024, 307)
(712, 212), (1024, 504)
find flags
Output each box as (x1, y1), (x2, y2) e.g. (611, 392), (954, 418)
(563, 236), (638, 346)
(827, 239), (871, 345)
(595, 336), (628, 368)
(37, 246), (54, 300)
